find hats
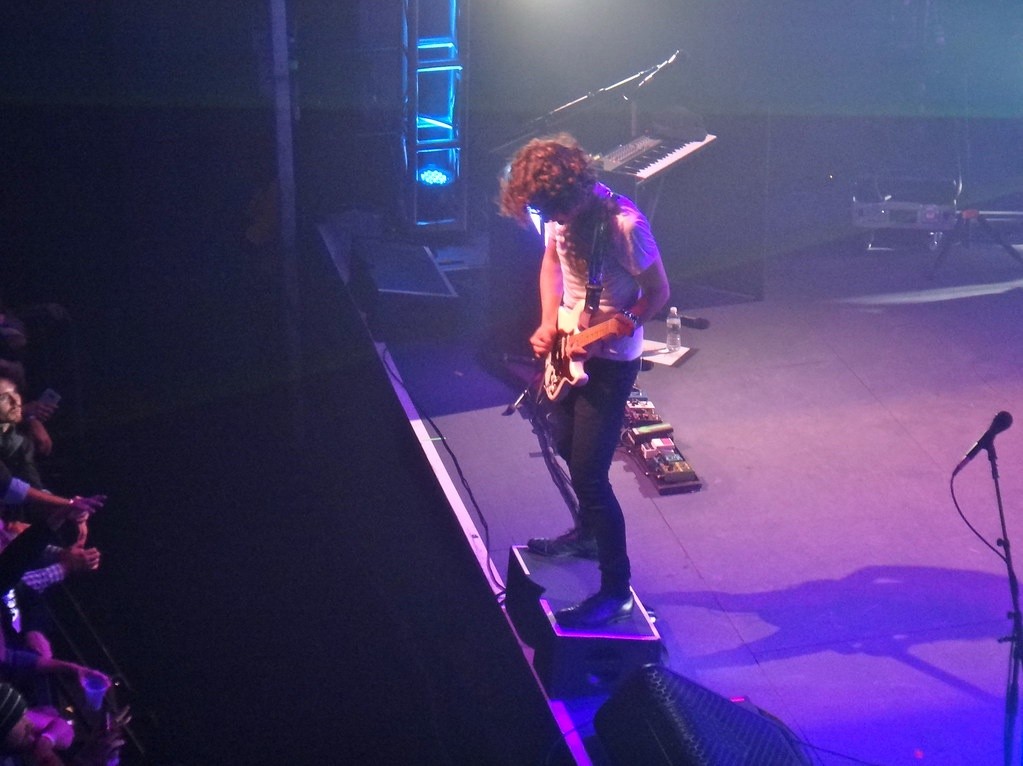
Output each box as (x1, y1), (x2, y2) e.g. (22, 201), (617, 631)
(0, 682), (27, 742)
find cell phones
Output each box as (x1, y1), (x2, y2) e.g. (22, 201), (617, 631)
(39, 388), (62, 421)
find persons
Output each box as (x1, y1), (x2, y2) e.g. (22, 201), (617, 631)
(0, 311), (126, 766)
(492, 132), (670, 631)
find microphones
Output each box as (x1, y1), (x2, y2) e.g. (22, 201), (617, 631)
(953, 409), (1015, 477)
(637, 46), (688, 90)
(591, 661), (808, 766)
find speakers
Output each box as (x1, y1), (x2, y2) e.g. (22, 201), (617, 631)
(350, 237), (460, 343)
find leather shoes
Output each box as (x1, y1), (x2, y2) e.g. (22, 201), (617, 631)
(527, 527), (599, 558)
(555, 592), (633, 627)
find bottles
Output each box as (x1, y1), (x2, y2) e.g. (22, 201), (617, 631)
(665, 307), (682, 352)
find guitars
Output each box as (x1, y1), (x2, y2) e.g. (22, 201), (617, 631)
(543, 296), (637, 402)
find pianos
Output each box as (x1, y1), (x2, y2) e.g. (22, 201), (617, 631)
(587, 124), (717, 329)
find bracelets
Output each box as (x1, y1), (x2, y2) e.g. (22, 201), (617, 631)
(621, 308), (639, 325)
